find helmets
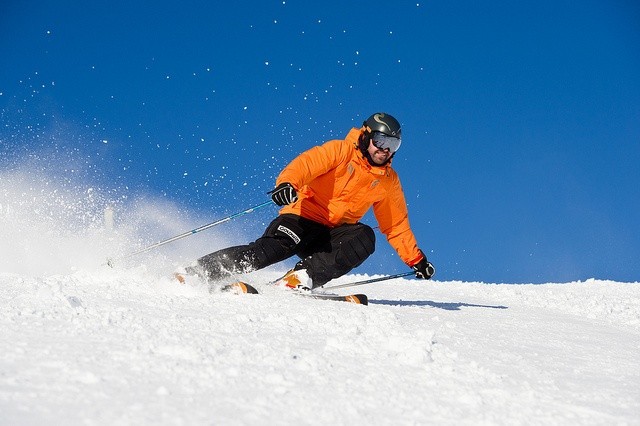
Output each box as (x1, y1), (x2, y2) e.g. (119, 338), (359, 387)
(360, 113), (402, 139)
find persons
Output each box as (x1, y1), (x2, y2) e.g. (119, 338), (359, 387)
(172, 111), (435, 295)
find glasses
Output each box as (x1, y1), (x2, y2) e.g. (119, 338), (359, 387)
(369, 130), (402, 153)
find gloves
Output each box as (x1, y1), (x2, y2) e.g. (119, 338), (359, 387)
(267, 182), (298, 207)
(410, 249), (436, 280)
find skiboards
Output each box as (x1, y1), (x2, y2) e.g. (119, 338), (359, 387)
(222, 282), (368, 305)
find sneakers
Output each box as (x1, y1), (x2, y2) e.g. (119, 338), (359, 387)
(271, 268), (313, 293)
(174, 266), (213, 294)
